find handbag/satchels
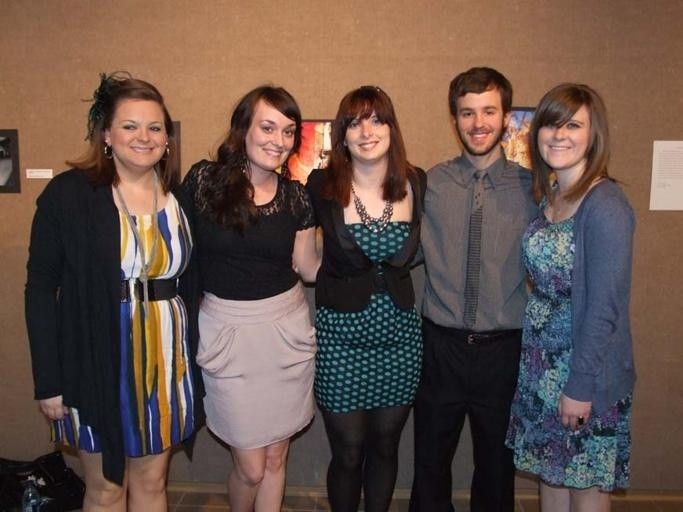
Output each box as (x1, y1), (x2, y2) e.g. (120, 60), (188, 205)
(0, 450), (86, 512)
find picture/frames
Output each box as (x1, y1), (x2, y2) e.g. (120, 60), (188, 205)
(285, 120), (336, 185)
(502, 107), (540, 169)
(168, 121), (181, 186)
(0, 129), (21, 193)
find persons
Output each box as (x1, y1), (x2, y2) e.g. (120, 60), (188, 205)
(24, 72), (206, 511)
(502, 83), (637, 511)
(302, 85), (428, 511)
(409, 68), (547, 512)
(175, 86), (324, 512)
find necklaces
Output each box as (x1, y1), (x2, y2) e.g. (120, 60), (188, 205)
(349, 182), (394, 233)
(553, 188), (566, 217)
(115, 170), (159, 314)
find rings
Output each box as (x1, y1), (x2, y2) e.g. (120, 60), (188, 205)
(578, 415), (583, 424)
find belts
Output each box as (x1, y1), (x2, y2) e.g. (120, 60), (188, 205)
(422, 315), (521, 345)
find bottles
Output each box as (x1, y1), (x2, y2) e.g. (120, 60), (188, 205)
(21, 480), (40, 512)
